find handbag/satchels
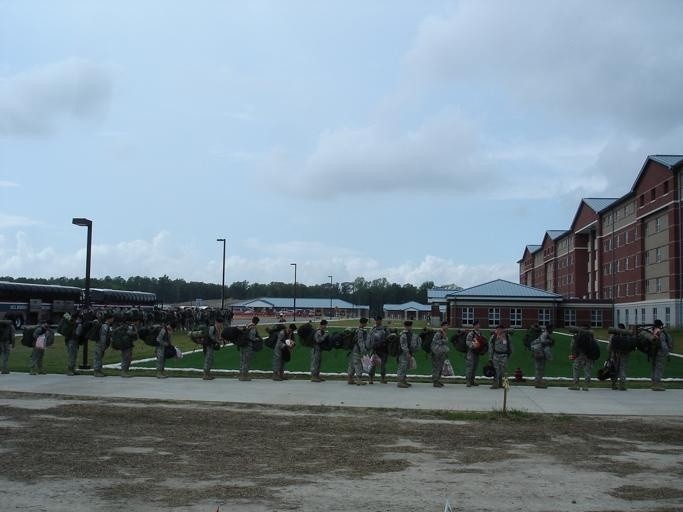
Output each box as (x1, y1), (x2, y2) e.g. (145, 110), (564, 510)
(407, 357), (418, 369)
(596, 360), (617, 381)
(441, 360), (454, 377)
(482, 360), (498, 377)
(360, 353), (383, 373)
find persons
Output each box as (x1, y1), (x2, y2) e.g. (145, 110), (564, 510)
(310, 320), (330, 382)
(531, 324), (553, 388)
(348, 318), (369, 385)
(608, 324), (628, 391)
(430, 322), (450, 386)
(273, 324), (297, 381)
(488, 325), (512, 388)
(368, 316), (388, 384)
(397, 321), (413, 387)
(465, 319), (480, 387)
(238, 317), (261, 381)
(651, 320), (670, 390)
(568, 334), (592, 391)
(0, 305), (234, 379)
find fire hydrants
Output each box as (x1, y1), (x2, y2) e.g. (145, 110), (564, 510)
(514, 368), (523, 382)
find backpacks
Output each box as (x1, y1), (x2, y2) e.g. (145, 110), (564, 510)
(280, 346), (291, 362)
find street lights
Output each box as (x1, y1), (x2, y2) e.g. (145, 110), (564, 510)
(327, 275), (332, 319)
(289, 263), (296, 321)
(71, 216), (92, 372)
(217, 238), (226, 309)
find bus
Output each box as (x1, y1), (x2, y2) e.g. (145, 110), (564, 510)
(82, 288), (156, 313)
(0, 279), (81, 329)
